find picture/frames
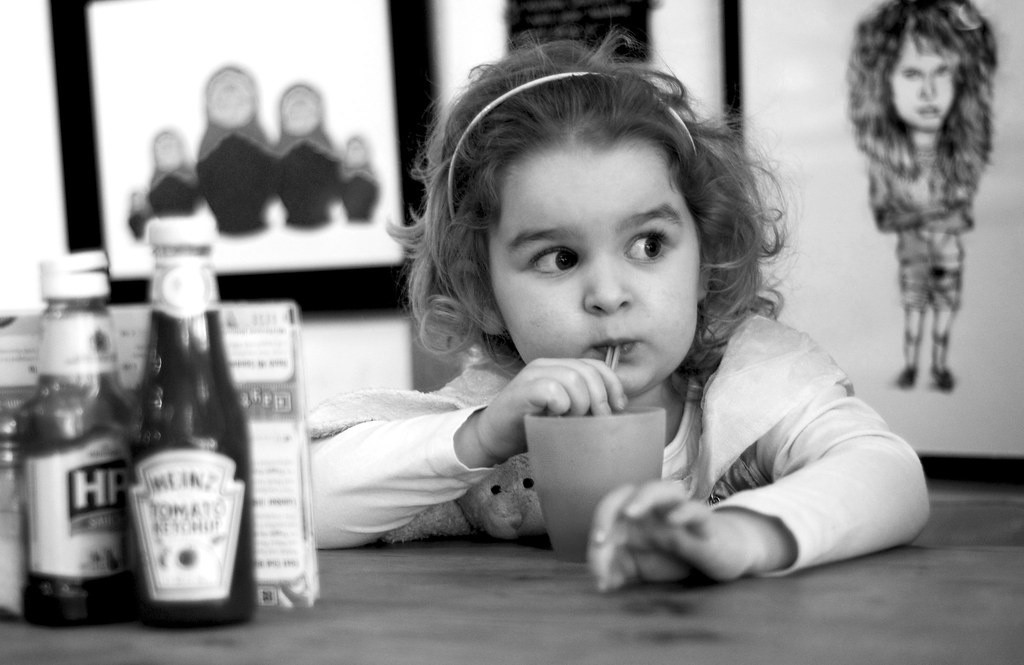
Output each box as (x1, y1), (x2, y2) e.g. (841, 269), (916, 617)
(720, 0), (1024, 482)
(48, 0), (445, 317)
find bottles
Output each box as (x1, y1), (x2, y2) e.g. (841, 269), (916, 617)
(24, 248), (130, 626)
(130, 217), (255, 627)
(1, 406), (24, 618)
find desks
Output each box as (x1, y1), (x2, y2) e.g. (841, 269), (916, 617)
(0, 543), (1024, 665)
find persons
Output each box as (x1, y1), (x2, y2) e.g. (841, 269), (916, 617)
(297, 29), (929, 592)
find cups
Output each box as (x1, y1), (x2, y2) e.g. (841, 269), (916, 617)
(524, 409), (667, 561)
